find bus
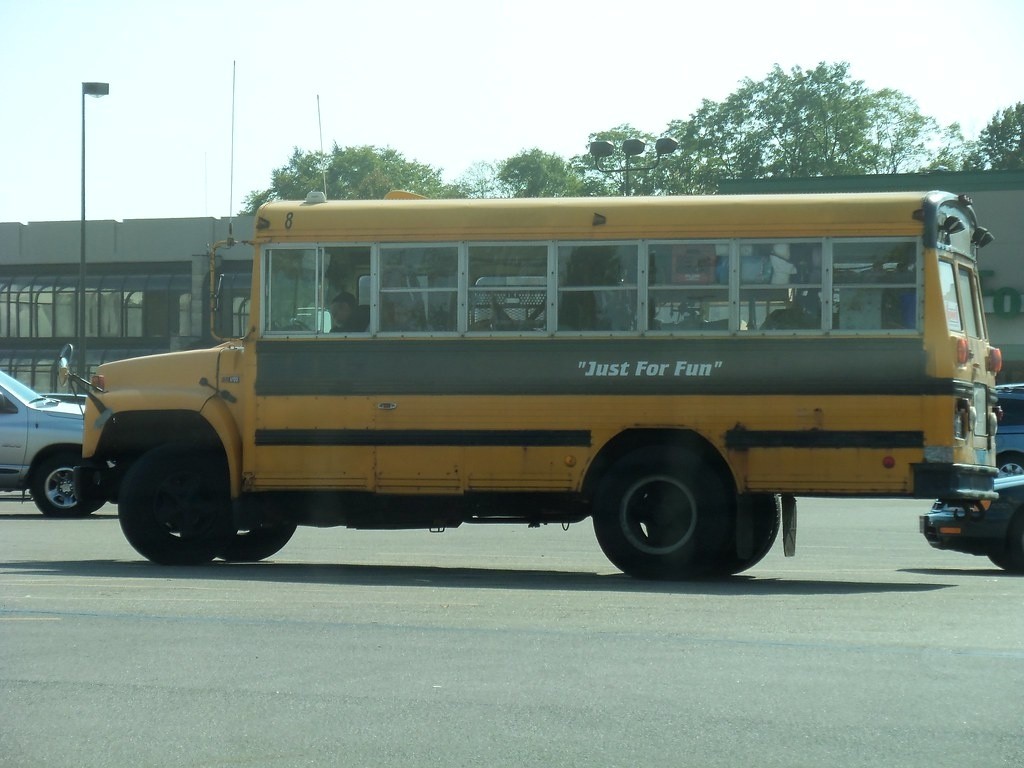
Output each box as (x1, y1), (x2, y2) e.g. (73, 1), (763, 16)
(59, 184), (1004, 585)
